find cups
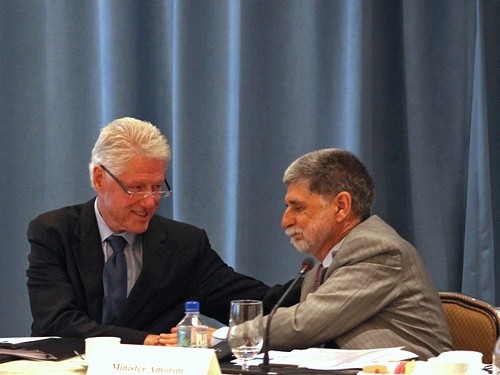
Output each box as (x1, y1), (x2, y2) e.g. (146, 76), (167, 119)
(85, 336), (121, 366)
(438, 350), (483, 374)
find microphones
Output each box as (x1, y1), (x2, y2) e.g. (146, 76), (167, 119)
(264, 257), (314, 370)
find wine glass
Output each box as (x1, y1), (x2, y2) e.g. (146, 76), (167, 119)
(228, 299), (265, 375)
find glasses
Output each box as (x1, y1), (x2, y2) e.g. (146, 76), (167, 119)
(101, 164), (172, 199)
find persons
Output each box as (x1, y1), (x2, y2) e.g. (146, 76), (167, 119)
(157, 147), (456, 361)
(25, 116), (306, 346)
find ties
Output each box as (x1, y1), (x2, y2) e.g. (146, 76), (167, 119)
(101, 235), (129, 327)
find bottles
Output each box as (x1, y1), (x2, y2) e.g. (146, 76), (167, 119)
(176, 301), (208, 348)
(492, 336), (500, 375)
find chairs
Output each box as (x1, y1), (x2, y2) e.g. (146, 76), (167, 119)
(438, 292), (500, 365)
